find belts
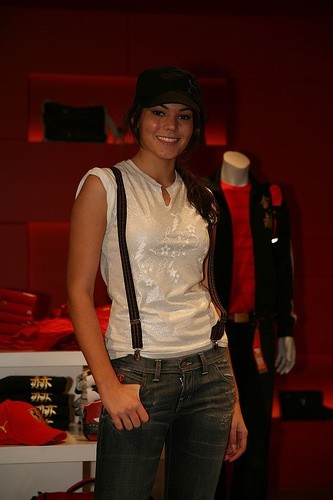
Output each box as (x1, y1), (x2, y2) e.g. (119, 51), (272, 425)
(226, 312), (258, 323)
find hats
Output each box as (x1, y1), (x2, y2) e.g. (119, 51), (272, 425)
(135, 66), (201, 115)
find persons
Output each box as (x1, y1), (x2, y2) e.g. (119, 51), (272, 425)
(66, 65), (250, 498)
(207, 152), (296, 498)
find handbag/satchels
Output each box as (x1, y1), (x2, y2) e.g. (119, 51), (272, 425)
(277, 391), (323, 422)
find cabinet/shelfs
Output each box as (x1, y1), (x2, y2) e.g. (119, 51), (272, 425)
(0, 351), (97, 500)
